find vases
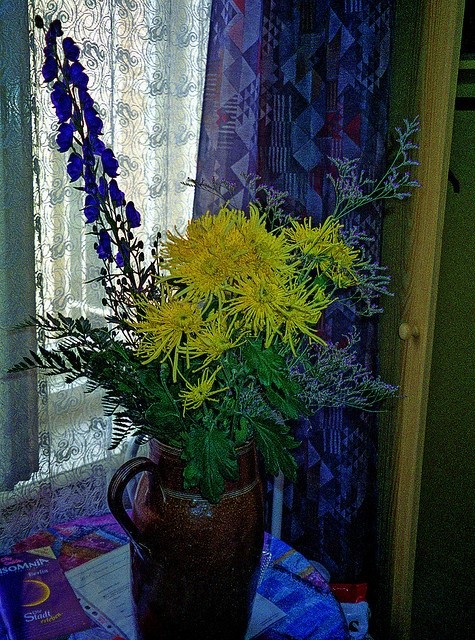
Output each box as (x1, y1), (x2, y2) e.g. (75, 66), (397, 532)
(106, 430), (265, 639)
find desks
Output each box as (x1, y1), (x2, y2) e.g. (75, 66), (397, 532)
(1, 508), (352, 640)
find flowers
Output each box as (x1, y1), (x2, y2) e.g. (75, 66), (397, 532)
(1, 14), (421, 507)
